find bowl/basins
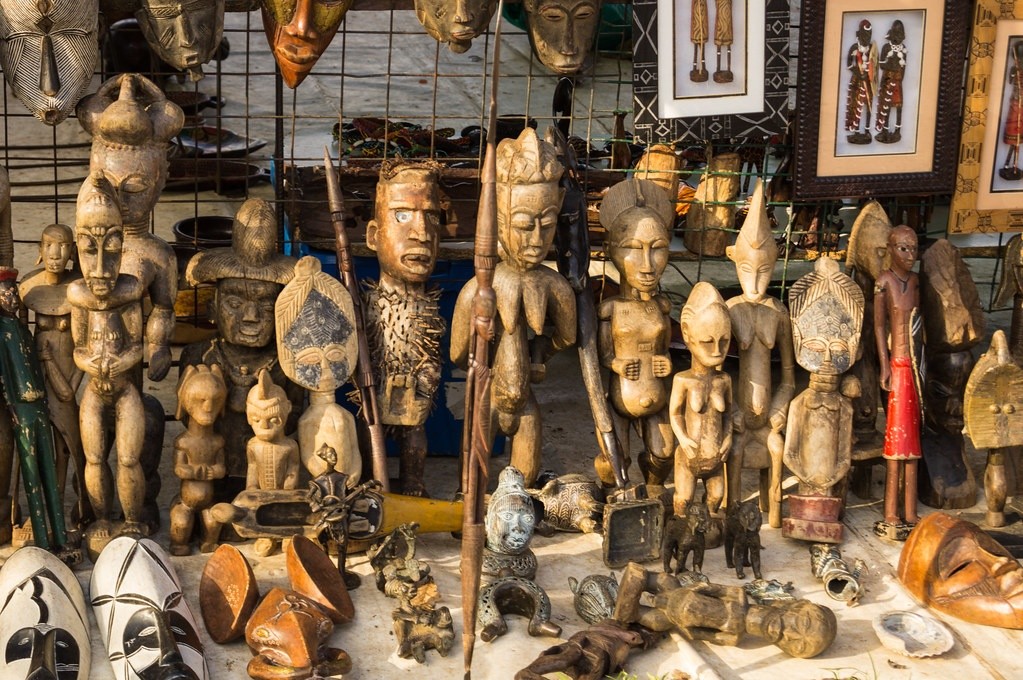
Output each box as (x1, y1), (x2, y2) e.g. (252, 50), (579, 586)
(496, 114), (538, 137)
(173, 216), (234, 265)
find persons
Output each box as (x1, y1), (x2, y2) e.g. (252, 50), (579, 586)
(1, 0), (1023, 658)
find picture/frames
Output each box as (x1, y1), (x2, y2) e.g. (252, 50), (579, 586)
(948, 0), (1023, 235)
(793, 0), (973, 203)
(656, 0), (765, 119)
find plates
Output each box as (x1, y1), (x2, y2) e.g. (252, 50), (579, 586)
(163, 91), (226, 115)
(170, 126), (267, 157)
(167, 160), (261, 188)
